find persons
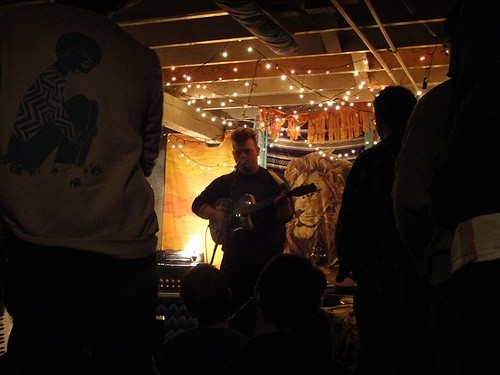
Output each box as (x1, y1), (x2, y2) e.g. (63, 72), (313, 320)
(191, 126), (295, 264)
(165, 263), (254, 375)
(0, 0), (164, 375)
(236, 256), (356, 375)
(334, 86), (418, 375)
(391, 1), (500, 375)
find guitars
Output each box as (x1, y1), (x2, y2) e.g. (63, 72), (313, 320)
(209, 183), (318, 245)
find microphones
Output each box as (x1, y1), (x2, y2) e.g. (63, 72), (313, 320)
(235, 159), (245, 168)
